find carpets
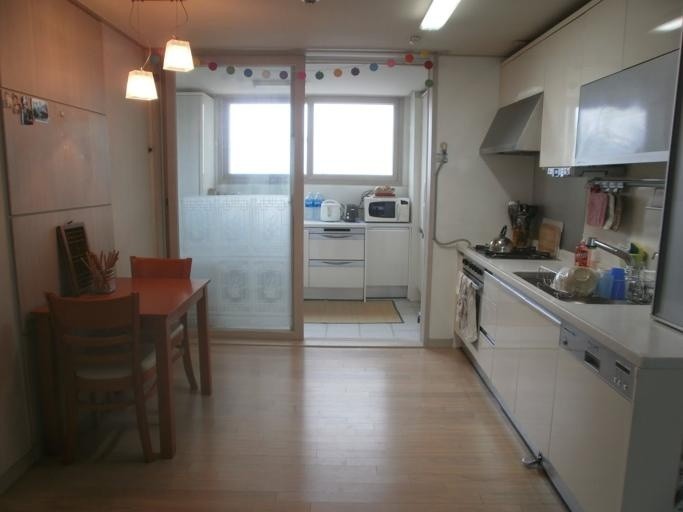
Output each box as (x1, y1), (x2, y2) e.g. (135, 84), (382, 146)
(303, 297), (403, 325)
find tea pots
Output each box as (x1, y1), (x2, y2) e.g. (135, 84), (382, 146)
(488, 224), (512, 254)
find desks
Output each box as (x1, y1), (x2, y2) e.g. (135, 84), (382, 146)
(27, 276), (211, 459)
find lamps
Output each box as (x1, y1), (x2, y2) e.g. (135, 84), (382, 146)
(158, 0), (195, 74)
(118, 2), (165, 107)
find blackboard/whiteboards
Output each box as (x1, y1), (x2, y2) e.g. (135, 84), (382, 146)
(59, 222), (91, 295)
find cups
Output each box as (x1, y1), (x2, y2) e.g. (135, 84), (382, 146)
(597, 264), (645, 302)
(512, 225), (534, 248)
(355, 217), (362, 222)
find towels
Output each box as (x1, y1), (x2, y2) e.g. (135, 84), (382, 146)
(450, 274), (476, 343)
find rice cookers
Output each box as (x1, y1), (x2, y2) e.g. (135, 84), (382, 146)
(319, 200), (341, 222)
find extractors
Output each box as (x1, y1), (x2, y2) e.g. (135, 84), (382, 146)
(480, 95), (540, 156)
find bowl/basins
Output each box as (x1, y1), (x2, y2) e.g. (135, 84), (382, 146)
(552, 265), (599, 300)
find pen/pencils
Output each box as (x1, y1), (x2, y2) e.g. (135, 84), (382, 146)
(81, 249), (120, 293)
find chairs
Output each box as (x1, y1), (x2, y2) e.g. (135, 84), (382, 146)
(43, 289), (162, 464)
(129, 256), (198, 393)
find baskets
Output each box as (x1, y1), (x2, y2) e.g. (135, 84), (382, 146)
(536, 266), (655, 301)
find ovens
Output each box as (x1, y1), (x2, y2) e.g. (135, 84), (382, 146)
(456, 266), (483, 380)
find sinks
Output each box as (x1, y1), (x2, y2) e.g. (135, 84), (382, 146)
(512, 269), (628, 302)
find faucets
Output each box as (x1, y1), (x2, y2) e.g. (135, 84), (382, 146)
(585, 235), (638, 298)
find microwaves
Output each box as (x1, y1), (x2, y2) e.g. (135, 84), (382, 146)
(363, 197), (410, 223)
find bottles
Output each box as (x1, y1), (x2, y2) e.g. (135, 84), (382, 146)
(313, 193), (322, 221)
(304, 191), (313, 221)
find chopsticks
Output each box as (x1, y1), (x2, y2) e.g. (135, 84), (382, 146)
(79, 248), (121, 293)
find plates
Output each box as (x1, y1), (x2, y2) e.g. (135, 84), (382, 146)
(374, 191), (395, 196)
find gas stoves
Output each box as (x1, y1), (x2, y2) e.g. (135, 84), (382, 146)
(468, 244), (552, 260)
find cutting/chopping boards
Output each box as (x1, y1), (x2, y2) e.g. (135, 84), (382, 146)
(538, 218), (564, 256)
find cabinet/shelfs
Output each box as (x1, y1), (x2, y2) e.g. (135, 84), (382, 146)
(498, 34), (544, 108)
(491, 277), (560, 472)
(539, 12), (580, 168)
(580, 1), (683, 86)
(366, 227), (409, 289)
